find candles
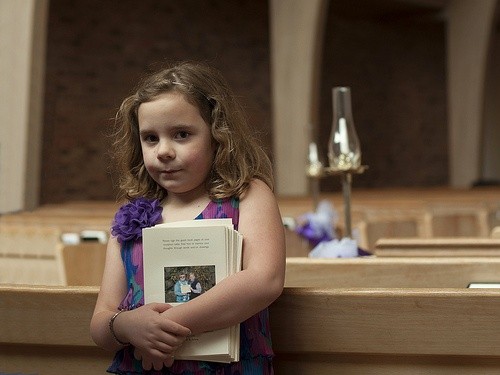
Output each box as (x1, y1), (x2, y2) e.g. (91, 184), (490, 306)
(332, 118), (349, 154)
(309, 142), (318, 164)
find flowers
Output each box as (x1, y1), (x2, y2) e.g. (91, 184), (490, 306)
(111, 198), (163, 241)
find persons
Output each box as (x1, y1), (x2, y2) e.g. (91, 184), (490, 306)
(89, 62), (285, 375)
(174, 273), (192, 302)
(187, 273), (202, 300)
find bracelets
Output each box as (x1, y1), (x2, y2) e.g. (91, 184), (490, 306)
(110, 308), (131, 346)
